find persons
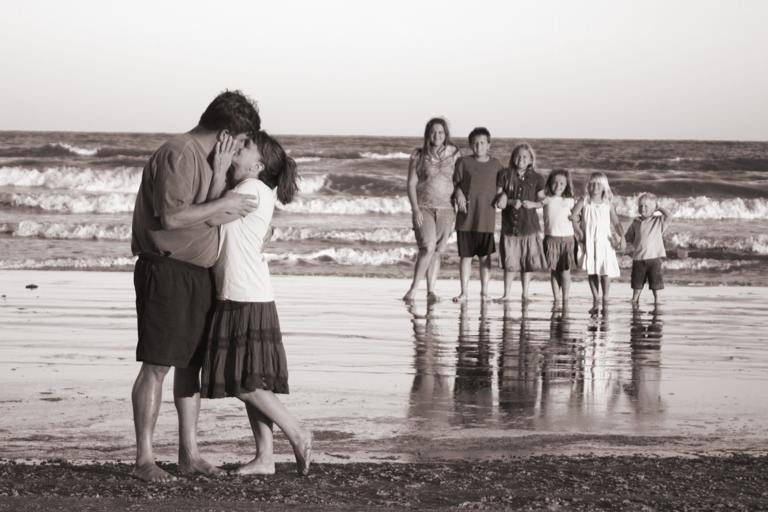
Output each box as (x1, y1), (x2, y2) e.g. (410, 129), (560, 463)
(567, 171), (626, 314)
(402, 302), (669, 434)
(490, 145), (548, 303)
(536, 168), (582, 314)
(623, 191), (674, 307)
(128, 86), (261, 484)
(451, 126), (504, 304)
(399, 115), (461, 303)
(200, 126), (314, 476)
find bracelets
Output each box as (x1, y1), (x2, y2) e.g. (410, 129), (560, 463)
(539, 200), (545, 208)
